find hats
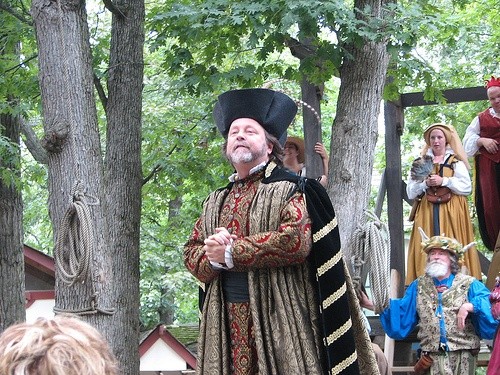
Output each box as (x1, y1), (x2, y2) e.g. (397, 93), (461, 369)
(212, 87), (298, 141)
(484, 75), (500, 89)
(424, 122), (452, 145)
(421, 236), (463, 259)
(286, 136), (305, 164)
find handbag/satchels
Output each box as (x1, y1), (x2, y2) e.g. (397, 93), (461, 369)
(426, 186), (451, 204)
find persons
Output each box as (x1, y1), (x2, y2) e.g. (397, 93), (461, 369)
(404, 123), (482, 291)
(356, 342), (392, 375)
(155, 88), (382, 375)
(283, 134), (331, 187)
(355, 226), (500, 375)
(461, 76), (500, 375)
(0, 312), (120, 375)
